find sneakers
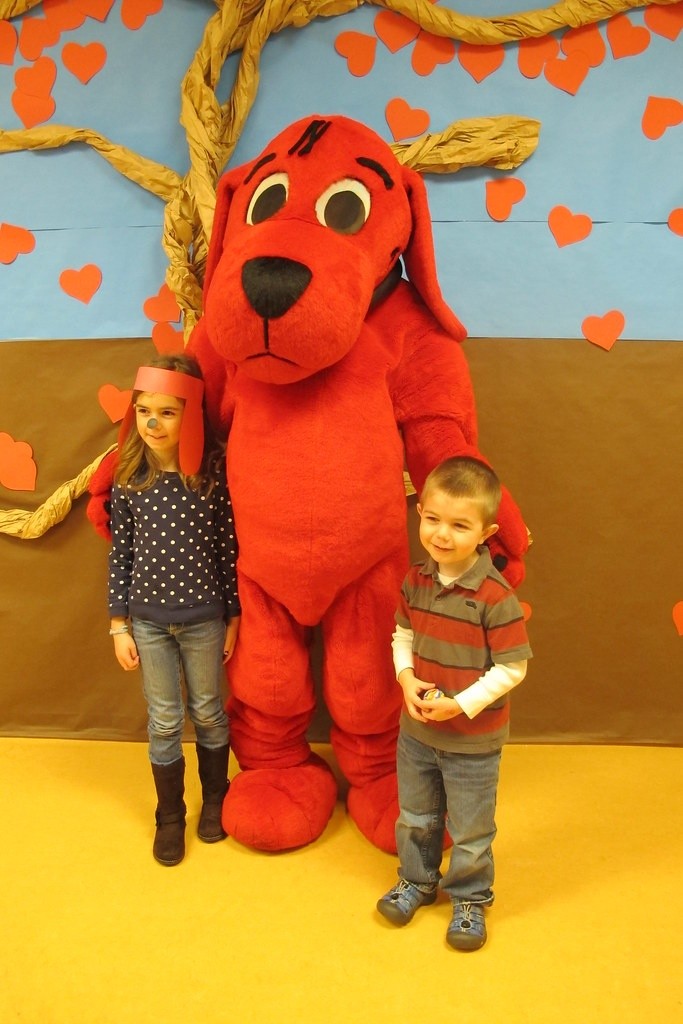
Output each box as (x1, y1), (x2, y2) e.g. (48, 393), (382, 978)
(377, 879), (438, 927)
(446, 903), (487, 950)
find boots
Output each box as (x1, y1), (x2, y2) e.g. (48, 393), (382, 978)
(195, 740), (231, 843)
(150, 756), (188, 866)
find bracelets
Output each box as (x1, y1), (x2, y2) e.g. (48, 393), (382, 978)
(109, 624), (128, 635)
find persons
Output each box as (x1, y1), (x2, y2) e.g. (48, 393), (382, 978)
(108, 355), (240, 866)
(377, 456), (533, 955)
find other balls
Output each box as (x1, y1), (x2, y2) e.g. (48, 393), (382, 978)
(423, 688), (444, 701)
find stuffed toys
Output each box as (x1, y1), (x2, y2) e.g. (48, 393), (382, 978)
(87, 116), (528, 855)
